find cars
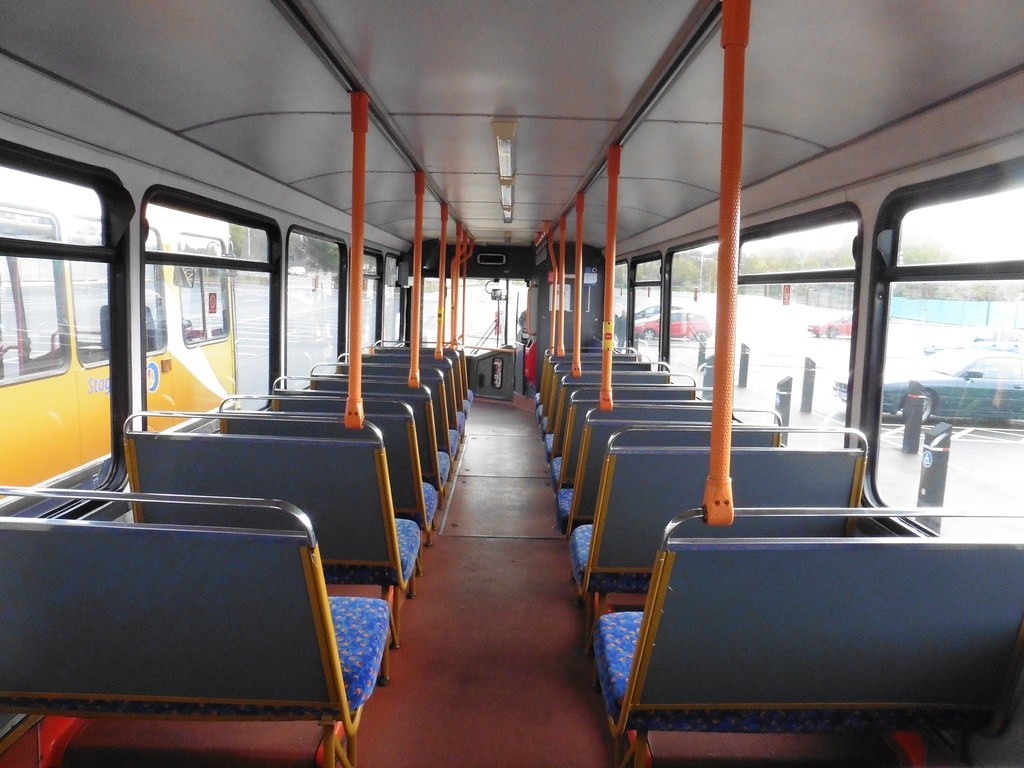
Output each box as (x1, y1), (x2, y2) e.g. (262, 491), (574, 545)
(829, 331), (1024, 421)
(626, 305), (711, 342)
(807, 318), (852, 339)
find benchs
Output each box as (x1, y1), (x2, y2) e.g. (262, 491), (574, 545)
(0, 340), (473, 768)
(534, 348), (1024, 768)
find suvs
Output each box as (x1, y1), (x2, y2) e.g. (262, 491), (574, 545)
(288, 267), (307, 276)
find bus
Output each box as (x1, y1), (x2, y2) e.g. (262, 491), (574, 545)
(0, 192), (240, 503)
